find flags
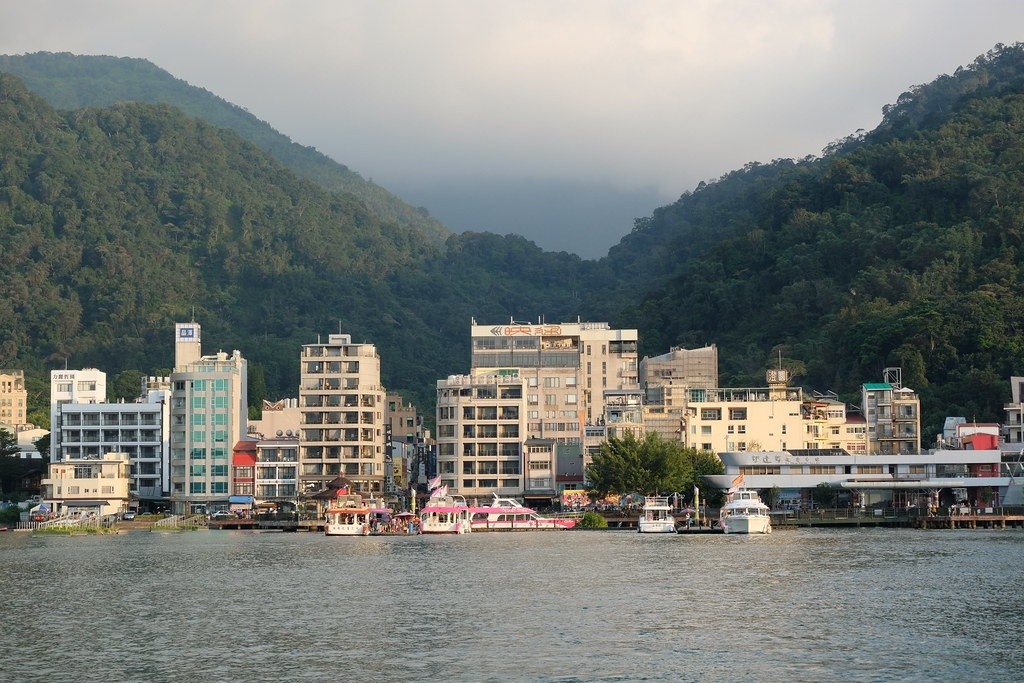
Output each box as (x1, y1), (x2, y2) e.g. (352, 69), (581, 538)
(428, 475), (447, 498)
(732, 474), (744, 487)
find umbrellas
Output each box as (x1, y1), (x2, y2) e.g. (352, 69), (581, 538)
(679, 508), (695, 513)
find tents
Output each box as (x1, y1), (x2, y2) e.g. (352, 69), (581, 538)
(30, 504), (50, 516)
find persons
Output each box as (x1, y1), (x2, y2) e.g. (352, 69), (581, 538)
(685, 512), (692, 527)
(32, 511), (53, 520)
(345, 515), (418, 533)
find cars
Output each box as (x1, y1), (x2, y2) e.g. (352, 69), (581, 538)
(212, 510), (239, 518)
(124, 511), (137, 520)
(143, 512), (153, 515)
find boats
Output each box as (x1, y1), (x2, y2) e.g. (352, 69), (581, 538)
(720, 481), (772, 535)
(323, 486), (420, 536)
(416, 491), (584, 535)
(638, 487), (675, 533)
